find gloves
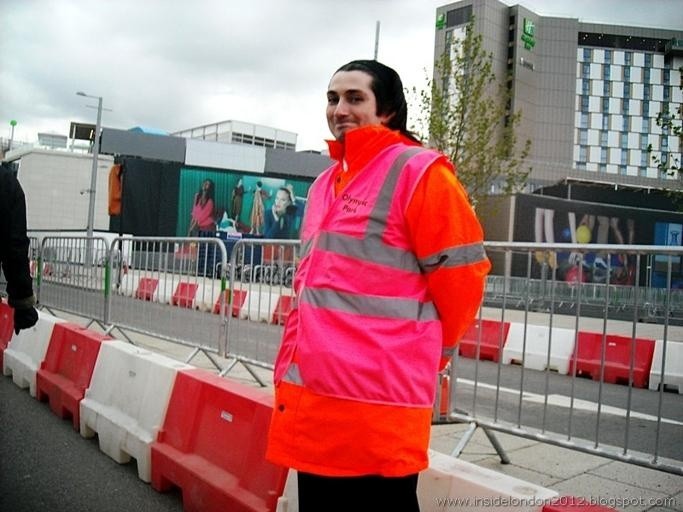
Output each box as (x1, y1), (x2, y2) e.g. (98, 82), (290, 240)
(13, 305), (38, 335)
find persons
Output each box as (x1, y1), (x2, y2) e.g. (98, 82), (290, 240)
(262, 187), (299, 239)
(265, 58), (494, 512)
(534, 207), (638, 277)
(188, 179), (215, 277)
(230, 179), (247, 227)
(1, 138), (38, 336)
(248, 181), (272, 235)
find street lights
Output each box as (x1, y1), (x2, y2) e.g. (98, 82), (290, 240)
(77, 92), (103, 269)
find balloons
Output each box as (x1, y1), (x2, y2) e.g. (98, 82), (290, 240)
(566, 267), (586, 289)
